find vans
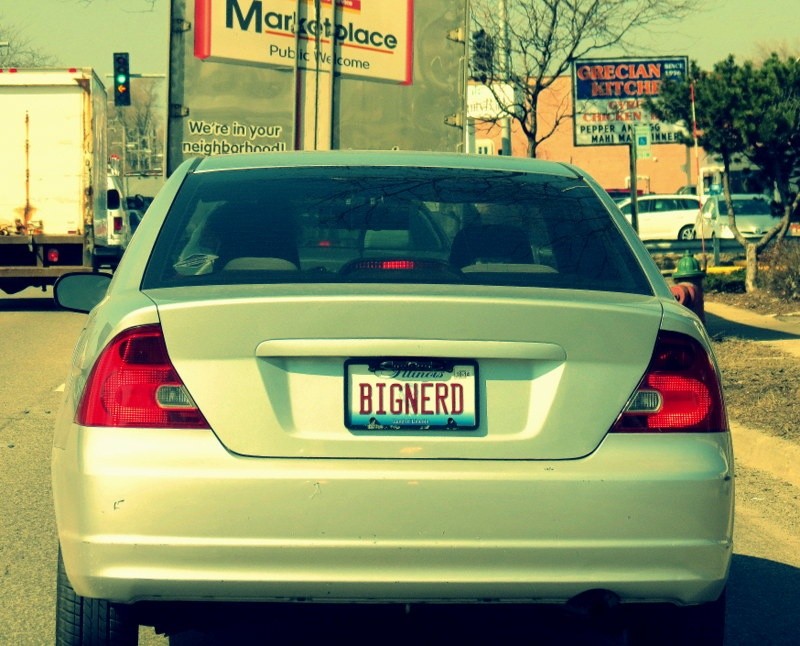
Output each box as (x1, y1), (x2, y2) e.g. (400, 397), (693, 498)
(91, 176), (132, 274)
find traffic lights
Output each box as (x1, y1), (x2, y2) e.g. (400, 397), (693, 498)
(470, 29), (493, 84)
(113, 51), (131, 107)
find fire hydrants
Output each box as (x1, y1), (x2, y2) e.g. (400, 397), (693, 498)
(670, 250), (707, 330)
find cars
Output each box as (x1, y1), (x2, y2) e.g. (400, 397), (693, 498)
(605, 185), (800, 241)
(52, 146), (735, 646)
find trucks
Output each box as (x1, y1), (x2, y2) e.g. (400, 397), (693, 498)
(0, 66), (110, 295)
